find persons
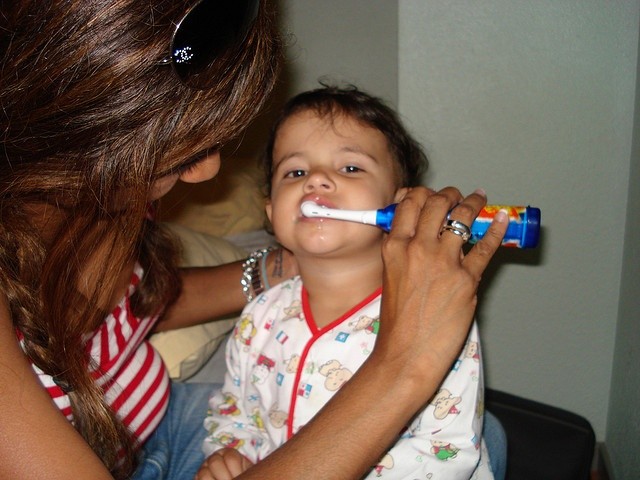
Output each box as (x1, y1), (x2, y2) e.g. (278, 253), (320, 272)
(195, 74), (495, 480)
(1, 1), (509, 480)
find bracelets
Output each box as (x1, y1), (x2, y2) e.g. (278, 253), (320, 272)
(240, 245), (278, 302)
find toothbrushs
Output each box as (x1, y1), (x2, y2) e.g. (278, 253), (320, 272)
(299, 199), (543, 251)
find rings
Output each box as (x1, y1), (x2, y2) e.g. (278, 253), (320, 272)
(443, 220), (471, 238)
(440, 227), (468, 243)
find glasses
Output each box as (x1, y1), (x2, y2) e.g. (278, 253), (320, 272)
(153, 0), (258, 84)
